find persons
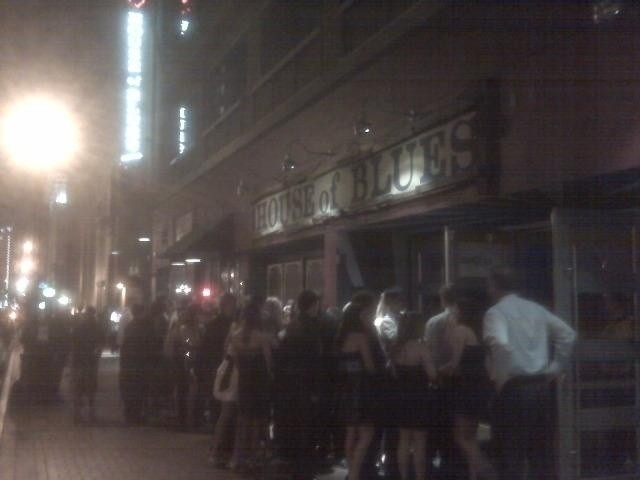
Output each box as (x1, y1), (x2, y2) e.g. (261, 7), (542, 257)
(70, 265), (579, 480)
